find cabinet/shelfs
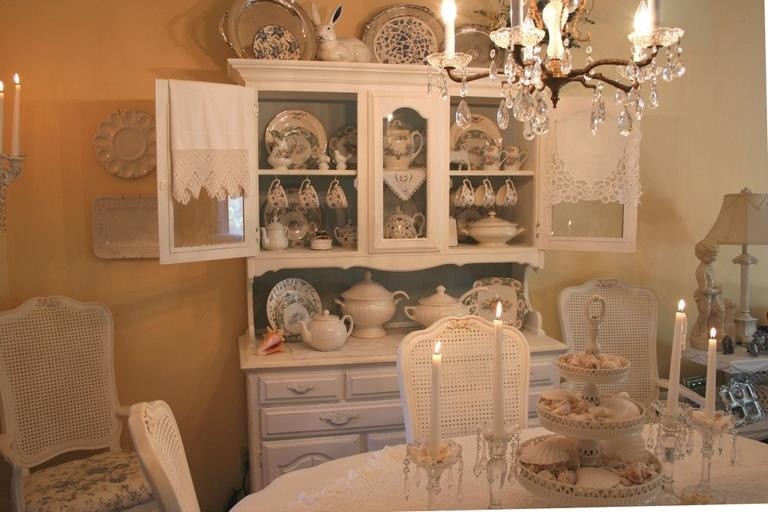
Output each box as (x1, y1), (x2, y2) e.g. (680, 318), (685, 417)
(667, 342), (768, 440)
(153, 59), (642, 277)
(255, 362), (559, 489)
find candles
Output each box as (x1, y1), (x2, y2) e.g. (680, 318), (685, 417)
(491, 302), (507, 426)
(667, 298), (689, 425)
(0, 85), (21, 157)
(706, 328), (716, 421)
(429, 337), (443, 455)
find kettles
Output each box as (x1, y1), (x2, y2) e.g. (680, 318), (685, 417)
(265, 133), (300, 171)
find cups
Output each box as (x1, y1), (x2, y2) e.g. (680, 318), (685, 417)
(453, 177), (519, 208)
(298, 179), (320, 209)
(266, 177), (290, 209)
(327, 179), (350, 209)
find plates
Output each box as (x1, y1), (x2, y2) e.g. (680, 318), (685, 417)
(355, 3), (446, 67)
(329, 122), (356, 170)
(263, 108), (328, 170)
(468, 275), (527, 331)
(450, 112), (503, 170)
(265, 278), (324, 343)
(251, 25), (301, 62)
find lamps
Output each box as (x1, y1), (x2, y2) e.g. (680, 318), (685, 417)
(702, 186), (768, 348)
(426, 0), (687, 142)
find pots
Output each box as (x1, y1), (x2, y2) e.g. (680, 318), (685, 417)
(334, 270), (411, 340)
(403, 284), (481, 331)
(460, 211), (526, 248)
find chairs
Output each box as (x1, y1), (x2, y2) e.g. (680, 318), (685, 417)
(127, 401), (201, 511)
(556, 279), (703, 421)
(1, 295), (156, 512)
(397, 315), (530, 445)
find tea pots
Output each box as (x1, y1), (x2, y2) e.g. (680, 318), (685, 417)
(501, 145), (529, 170)
(482, 144), (509, 171)
(259, 215), (291, 251)
(298, 309), (353, 353)
